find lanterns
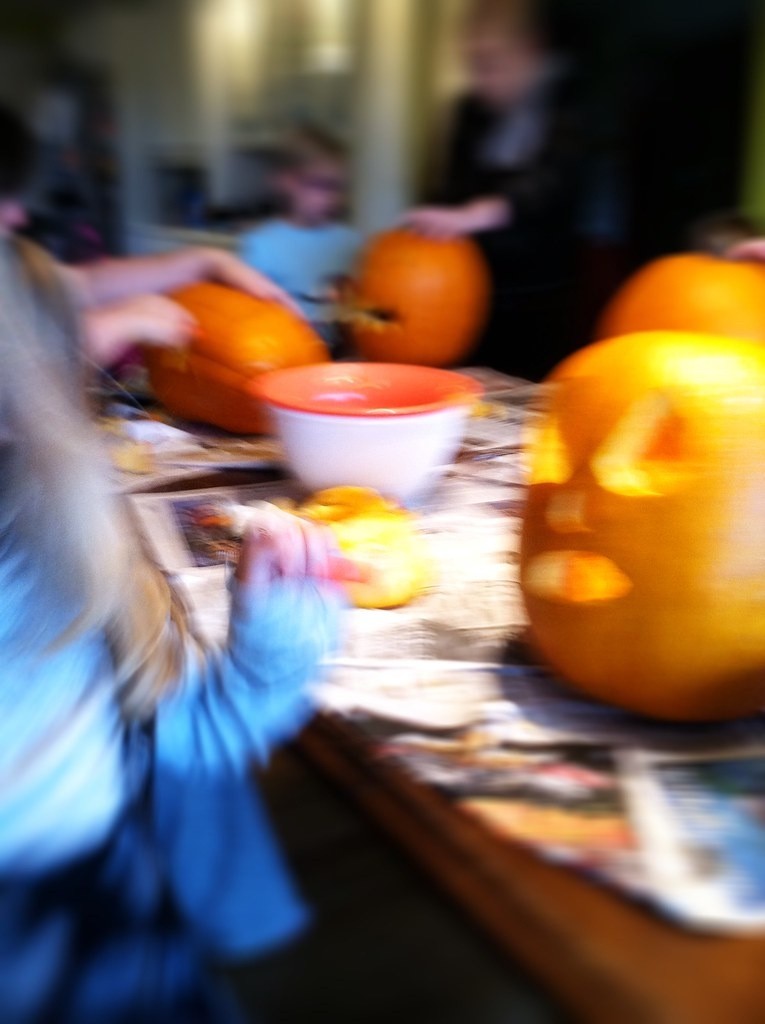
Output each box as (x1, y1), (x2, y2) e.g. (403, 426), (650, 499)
(336, 222), (488, 367)
(516, 331), (765, 722)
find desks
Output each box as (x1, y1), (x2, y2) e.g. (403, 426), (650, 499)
(72, 354), (765, 1024)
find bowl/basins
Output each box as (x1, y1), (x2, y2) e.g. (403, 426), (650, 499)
(246, 362), (482, 505)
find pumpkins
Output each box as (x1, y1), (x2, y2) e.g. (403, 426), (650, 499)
(594, 251), (765, 354)
(151, 284), (329, 436)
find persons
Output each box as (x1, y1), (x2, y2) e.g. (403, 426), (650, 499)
(238, 129), (365, 359)
(0, 108), (344, 1024)
(404, 0), (615, 384)
